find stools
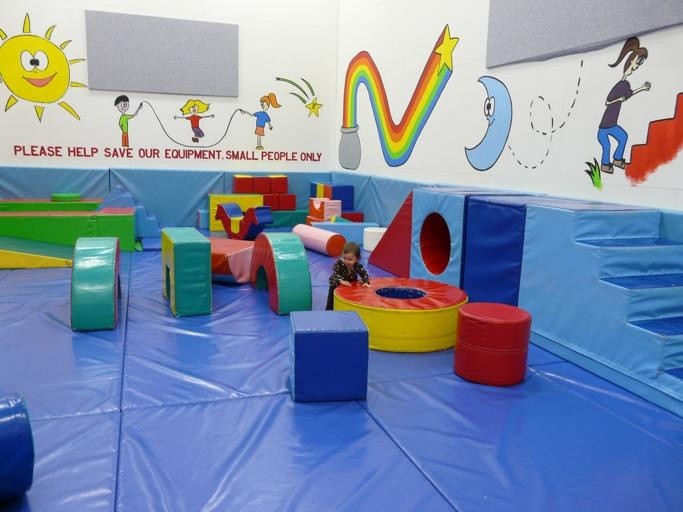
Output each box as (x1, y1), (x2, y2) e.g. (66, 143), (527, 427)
(288, 310), (368, 401)
(453, 301), (532, 386)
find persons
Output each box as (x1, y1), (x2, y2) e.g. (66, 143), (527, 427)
(173, 99), (214, 143)
(242, 93), (282, 150)
(114, 94), (142, 148)
(596, 35), (652, 175)
(325, 242), (372, 311)
